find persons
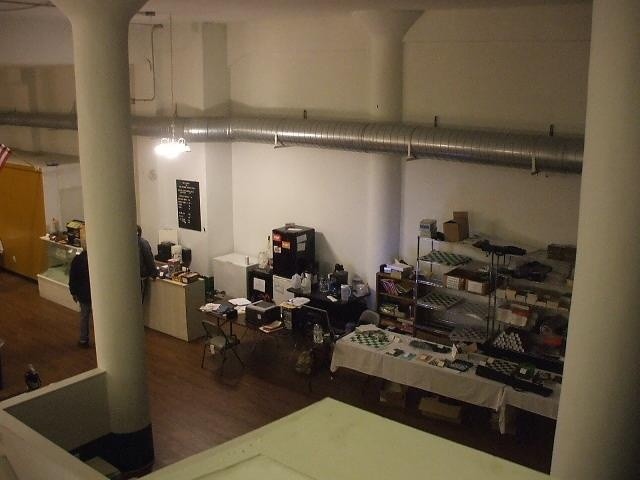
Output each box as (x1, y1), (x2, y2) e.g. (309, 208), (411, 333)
(69, 240), (93, 347)
(138, 225), (158, 304)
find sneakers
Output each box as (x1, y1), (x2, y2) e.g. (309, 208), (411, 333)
(77, 339), (90, 349)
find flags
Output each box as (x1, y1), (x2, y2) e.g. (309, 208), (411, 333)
(0, 144), (12, 172)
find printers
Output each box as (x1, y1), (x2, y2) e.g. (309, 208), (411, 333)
(246, 300), (280, 326)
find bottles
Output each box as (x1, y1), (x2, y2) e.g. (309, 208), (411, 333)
(52, 216), (59, 233)
(205, 280), (214, 303)
(329, 264), (349, 299)
(258, 235), (272, 270)
(290, 272), (367, 302)
(313, 323), (323, 344)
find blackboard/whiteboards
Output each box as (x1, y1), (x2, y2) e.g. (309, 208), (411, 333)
(176, 179), (201, 231)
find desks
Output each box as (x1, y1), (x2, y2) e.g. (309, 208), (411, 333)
(496, 367), (563, 436)
(286, 285), (372, 333)
(195, 297), (287, 355)
(330, 325), (518, 411)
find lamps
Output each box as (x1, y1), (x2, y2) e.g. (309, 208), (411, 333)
(153, 113), (192, 155)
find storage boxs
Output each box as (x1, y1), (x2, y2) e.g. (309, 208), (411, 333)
(419, 396), (466, 425)
(378, 378), (407, 410)
(443, 211), (470, 243)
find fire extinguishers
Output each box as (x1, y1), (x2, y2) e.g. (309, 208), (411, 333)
(24, 364), (41, 390)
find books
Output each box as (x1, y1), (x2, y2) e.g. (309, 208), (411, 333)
(378, 259), (413, 333)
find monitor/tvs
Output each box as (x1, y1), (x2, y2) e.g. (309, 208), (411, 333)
(299, 304), (330, 335)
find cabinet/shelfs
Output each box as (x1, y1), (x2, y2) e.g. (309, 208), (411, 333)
(36, 234), (84, 313)
(0, 153), (83, 282)
(375, 234), (576, 376)
(210, 223), (321, 330)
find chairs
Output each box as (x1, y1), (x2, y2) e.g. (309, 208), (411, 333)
(199, 320), (249, 375)
(353, 308), (380, 330)
